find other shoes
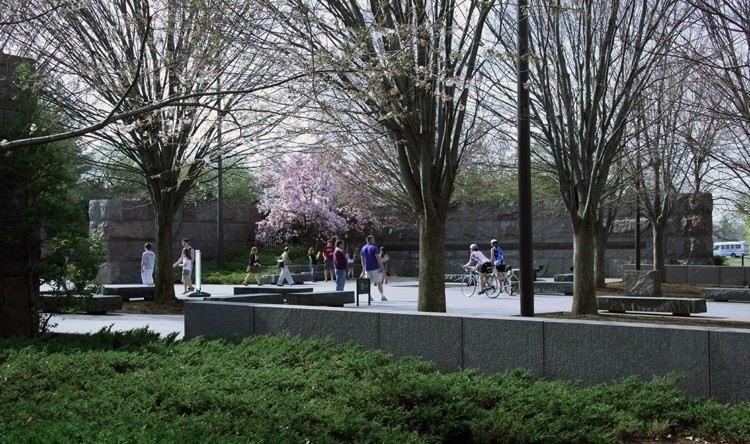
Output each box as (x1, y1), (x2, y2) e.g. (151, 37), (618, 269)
(243, 282), (248, 286)
(478, 291), (484, 294)
(370, 298), (374, 301)
(258, 283), (264, 286)
(382, 297), (387, 301)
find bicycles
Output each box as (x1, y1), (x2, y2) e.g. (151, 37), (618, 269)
(460, 265), (520, 298)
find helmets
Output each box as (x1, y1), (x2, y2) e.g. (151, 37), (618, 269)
(490, 239), (497, 244)
(470, 243), (478, 250)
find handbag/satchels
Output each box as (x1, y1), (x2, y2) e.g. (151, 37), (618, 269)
(246, 266), (251, 272)
(277, 260), (284, 268)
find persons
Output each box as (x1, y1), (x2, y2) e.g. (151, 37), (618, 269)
(173, 238), (195, 294)
(322, 239), (356, 292)
(489, 239), (513, 295)
(140, 243), (156, 284)
(360, 235), (390, 301)
(276, 246), (295, 286)
(308, 247), (321, 283)
(463, 243), (492, 295)
(242, 246), (263, 286)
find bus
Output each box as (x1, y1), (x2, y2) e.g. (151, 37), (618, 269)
(713, 241), (749, 258)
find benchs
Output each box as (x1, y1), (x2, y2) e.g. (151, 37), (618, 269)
(444, 274), (478, 287)
(595, 296), (707, 317)
(204, 292), (284, 305)
(534, 281), (574, 296)
(41, 289), (92, 295)
(268, 273), (313, 285)
(702, 288), (750, 303)
(302, 270), (330, 281)
(286, 290), (355, 307)
(103, 284), (155, 304)
(37, 294), (122, 316)
(233, 287), (313, 301)
